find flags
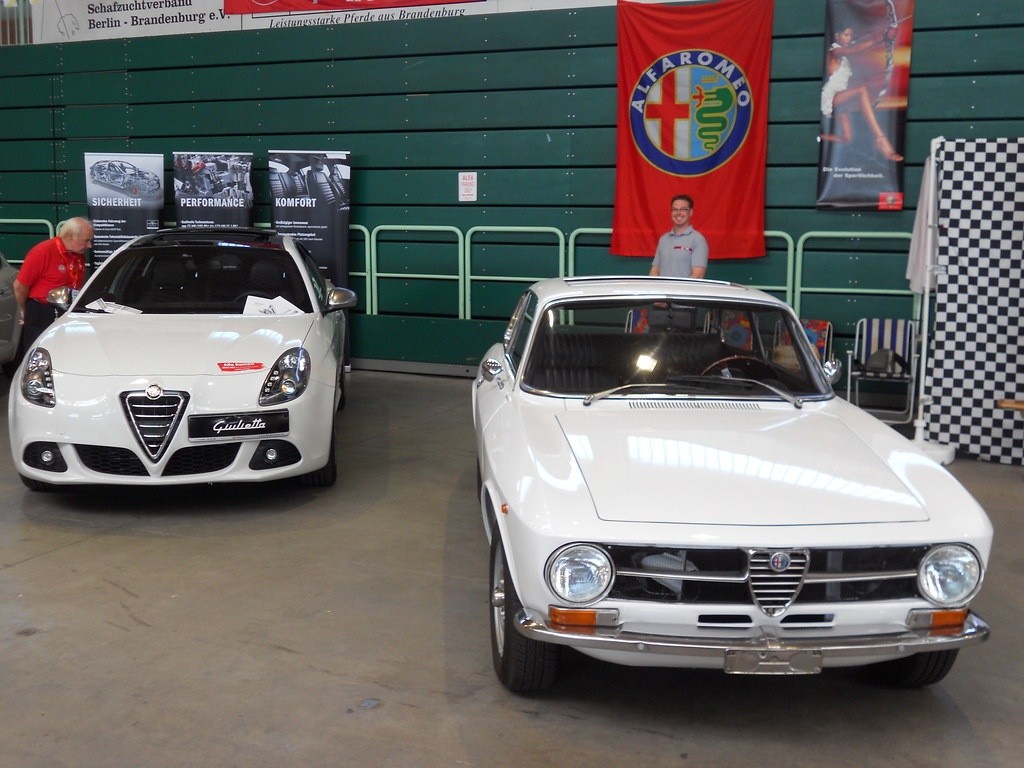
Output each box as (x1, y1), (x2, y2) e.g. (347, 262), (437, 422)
(609, 0), (775, 260)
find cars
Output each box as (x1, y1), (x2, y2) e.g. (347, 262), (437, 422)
(472, 276), (994, 696)
(8, 228), (358, 492)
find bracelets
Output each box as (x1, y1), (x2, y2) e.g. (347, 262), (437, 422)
(884, 32), (889, 42)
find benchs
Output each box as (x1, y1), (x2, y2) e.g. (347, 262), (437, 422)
(540, 332), (723, 394)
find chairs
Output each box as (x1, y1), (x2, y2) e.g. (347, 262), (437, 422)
(773, 317), (834, 376)
(238, 260), (278, 304)
(702, 309), (759, 355)
(622, 309), (649, 332)
(845, 317), (922, 426)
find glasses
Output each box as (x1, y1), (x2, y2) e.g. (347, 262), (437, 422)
(671, 207), (690, 211)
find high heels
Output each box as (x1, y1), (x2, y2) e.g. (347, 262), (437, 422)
(873, 135), (904, 163)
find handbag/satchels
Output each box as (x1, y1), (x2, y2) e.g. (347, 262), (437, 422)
(865, 348), (907, 374)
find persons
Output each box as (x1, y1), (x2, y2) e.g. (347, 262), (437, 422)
(648, 195), (710, 279)
(818, 23), (904, 163)
(12, 216), (95, 352)
